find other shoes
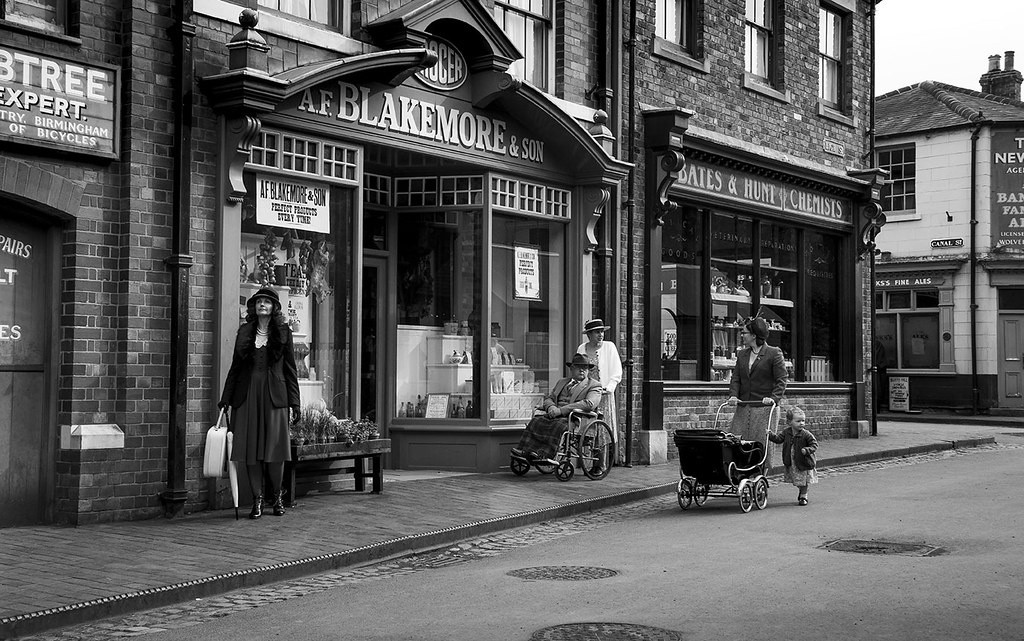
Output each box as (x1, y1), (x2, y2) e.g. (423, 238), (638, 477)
(529, 451), (541, 460)
(798, 490), (808, 505)
(249, 494), (265, 518)
(271, 490), (288, 515)
(584, 468), (603, 476)
(512, 447), (526, 457)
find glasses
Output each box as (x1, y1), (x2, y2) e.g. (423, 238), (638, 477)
(572, 365), (589, 369)
(741, 330), (750, 334)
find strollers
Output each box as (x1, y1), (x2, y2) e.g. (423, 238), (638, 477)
(672, 400), (776, 513)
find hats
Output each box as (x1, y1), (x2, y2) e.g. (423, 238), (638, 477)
(581, 319), (611, 334)
(247, 287), (282, 311)
(566, 353), (595, 368)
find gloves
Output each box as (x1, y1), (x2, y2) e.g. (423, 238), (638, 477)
(606, 380), (618, 394)
(292, 406), (301, 425)
(218, 400), (229, 413)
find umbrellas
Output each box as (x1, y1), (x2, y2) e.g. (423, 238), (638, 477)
(224, 410), (239, 519)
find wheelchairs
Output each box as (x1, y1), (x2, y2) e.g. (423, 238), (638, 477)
(509, 407), (615, 482)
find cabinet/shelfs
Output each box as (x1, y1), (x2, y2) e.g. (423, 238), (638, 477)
(710, 259), (798, 382)
(240, 233), (324, 404)
(425, 334), (545, 419)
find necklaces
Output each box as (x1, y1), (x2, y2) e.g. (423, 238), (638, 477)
(752, 349), (758, 355)
(256, 327), (268, 336)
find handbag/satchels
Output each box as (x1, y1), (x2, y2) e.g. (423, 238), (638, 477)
(203, 407), (228, 479)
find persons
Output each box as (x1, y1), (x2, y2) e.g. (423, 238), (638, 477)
(218, 287), (301, 519)
(727, 317), (789, 476)
(511, 353), (603, 466)
(576, 319), (623, 476)
(765, 406), (818, 505)
